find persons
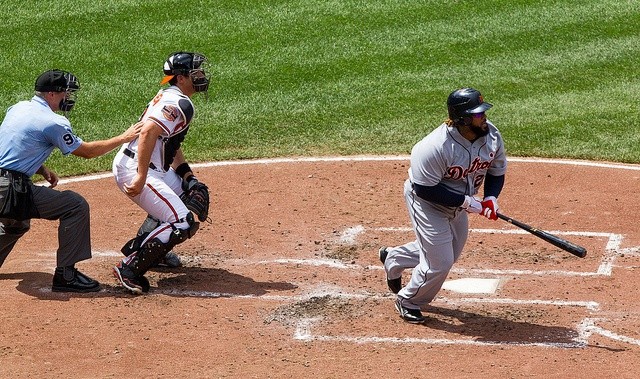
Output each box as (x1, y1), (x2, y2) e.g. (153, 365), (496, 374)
(378, 87), (507, 324)
(112, 51), (212, 295)
(0, 69), (146, 293)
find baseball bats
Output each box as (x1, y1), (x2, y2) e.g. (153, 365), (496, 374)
(496, 213), (587, 259)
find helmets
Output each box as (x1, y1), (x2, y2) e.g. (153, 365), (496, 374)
(447, 87), (493, 124)
(160, 51), (213, 95)
(35, 68), (81, 118)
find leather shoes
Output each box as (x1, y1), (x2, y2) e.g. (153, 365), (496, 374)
(52, 266), (101, 294)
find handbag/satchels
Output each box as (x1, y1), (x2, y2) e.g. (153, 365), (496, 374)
(1, 173), (41, 221)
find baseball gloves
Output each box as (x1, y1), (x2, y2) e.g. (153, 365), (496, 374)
(179, 181), (209, 222)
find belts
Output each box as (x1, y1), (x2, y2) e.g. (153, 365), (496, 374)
(0, 168), (31, 182)
(123, 147), (161, 172)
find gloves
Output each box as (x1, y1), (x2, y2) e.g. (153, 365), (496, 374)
(466, 196), (484, 215)
(483, 196), (500, 221)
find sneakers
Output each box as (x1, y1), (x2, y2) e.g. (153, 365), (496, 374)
(111, 260), (144, 294)
(378, 245), (403, 295)
(393, 298), (426, 324)
(159, 253), (180, 268)
